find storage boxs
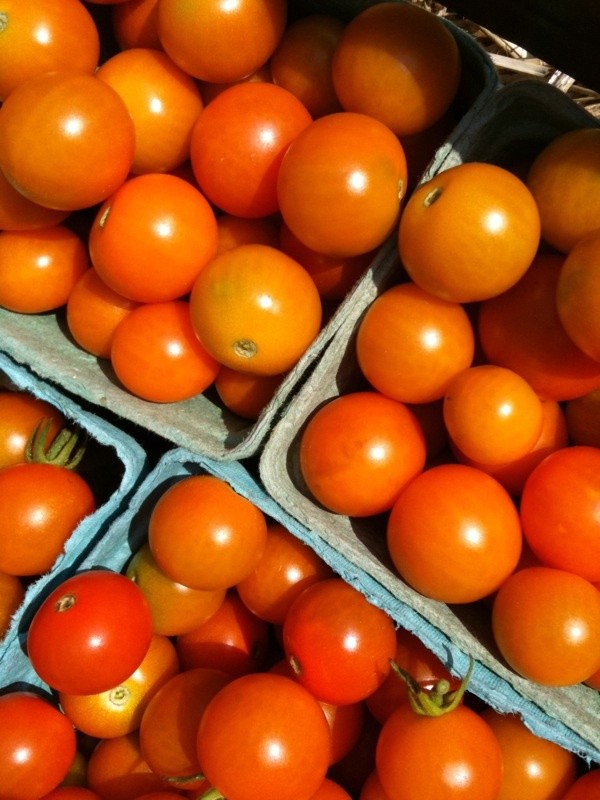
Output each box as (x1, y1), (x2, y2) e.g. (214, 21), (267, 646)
(1, 0), (600, 763)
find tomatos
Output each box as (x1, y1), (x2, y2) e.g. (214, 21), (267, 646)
(0, 0), (600, 799)
